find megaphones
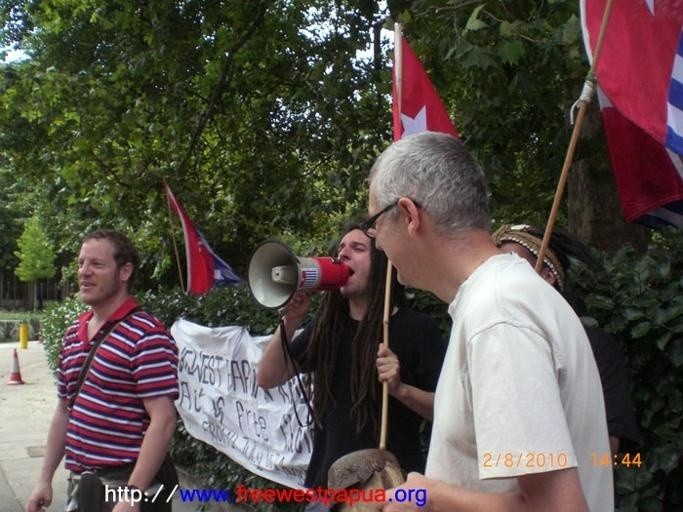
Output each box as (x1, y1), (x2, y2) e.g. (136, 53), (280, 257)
(247, 240), (350, 309)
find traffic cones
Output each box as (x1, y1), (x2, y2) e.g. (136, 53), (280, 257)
(7, 347), (25, 385)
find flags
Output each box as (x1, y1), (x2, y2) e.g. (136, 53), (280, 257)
(391, 37), (460, 141)
(167, 188), (241, 299)
(580, 0), (683, 226)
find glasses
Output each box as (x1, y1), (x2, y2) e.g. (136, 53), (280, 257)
(360, 198), (422, 239)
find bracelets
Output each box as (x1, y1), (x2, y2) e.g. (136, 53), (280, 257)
(119, 484), (147, 503)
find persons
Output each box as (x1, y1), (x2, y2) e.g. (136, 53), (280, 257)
(26, 232), (180, 512)
(255, 225), (447, 512)
(360, 132), (615, 512)
(491, 221), (643, 464)
